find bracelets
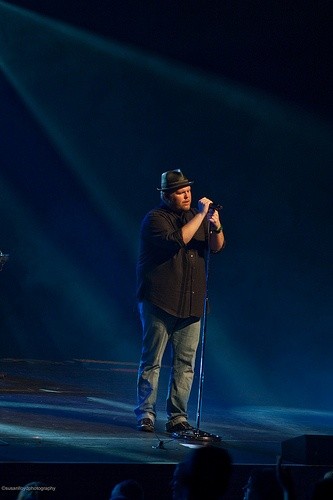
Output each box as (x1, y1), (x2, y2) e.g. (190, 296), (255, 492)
(212, 226), (223, 234)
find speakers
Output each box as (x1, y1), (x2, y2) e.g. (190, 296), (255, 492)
(281, 434), (333, 465)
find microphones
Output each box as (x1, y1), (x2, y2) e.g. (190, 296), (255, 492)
(209, 203), (224, 211)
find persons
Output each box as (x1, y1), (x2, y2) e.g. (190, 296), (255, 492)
(17, 445), (333, 500)
(137, 170), (225, 436)
